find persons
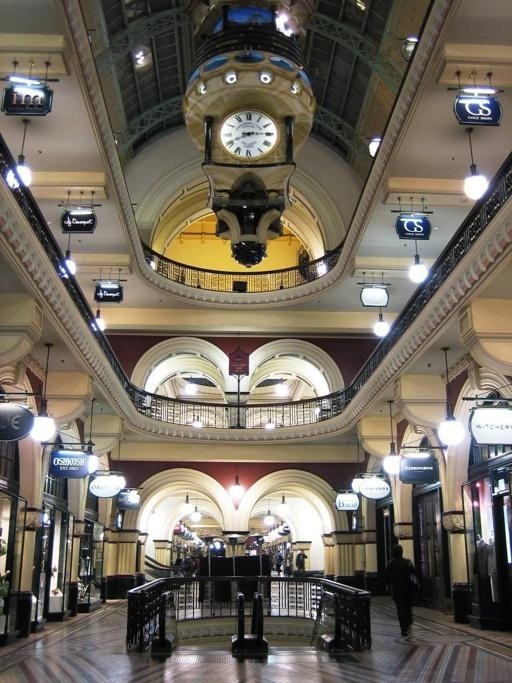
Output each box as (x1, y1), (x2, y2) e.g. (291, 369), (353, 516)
(174, 551), (202, 577)
(273, 549), (283, 576)
(295, 548), (308, 577)
(383, 543), (425, 635)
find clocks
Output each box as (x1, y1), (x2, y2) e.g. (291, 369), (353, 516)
(216, 104), (284, 163)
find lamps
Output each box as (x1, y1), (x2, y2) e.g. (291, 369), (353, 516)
(28, 342), (100, 475)
(372, 128), (491, 340)
(7, 118), (80, 276)
(381, 346), (466, 476)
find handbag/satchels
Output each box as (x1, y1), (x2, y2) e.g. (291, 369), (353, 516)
(278, 555), (283, 561)
(409, 574), (423, 593)
